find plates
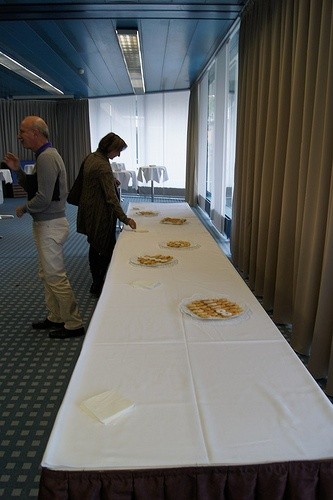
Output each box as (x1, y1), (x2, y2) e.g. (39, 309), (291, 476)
(160, 216), (189, 224)
(135, 211), (159, 216)
(179, 294), (248, 320)
(129, 254), (178, 267)
(159, 239), (199, 249)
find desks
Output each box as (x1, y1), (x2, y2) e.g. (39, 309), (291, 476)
(113, 170), (138, 203)
(136, 165), (168, 203)
(39, 202), (333, 500)
(0, 169), (14, 220)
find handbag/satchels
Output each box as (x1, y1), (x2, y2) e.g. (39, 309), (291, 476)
(25, 174), (61, 202)
(66, 153), (93, 206)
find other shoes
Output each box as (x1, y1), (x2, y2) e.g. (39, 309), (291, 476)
(90, 284), (101, 294)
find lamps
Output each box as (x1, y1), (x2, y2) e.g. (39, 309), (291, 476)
(0, 51), (65, 96)
(115, 29), (145, 95)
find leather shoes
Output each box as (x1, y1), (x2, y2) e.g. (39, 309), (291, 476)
(49, 327), (86, 338)
(32, 319), (65, 329)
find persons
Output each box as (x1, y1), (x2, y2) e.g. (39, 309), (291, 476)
(4, 116), (85, 337)
(76, 133), (136, 294)
